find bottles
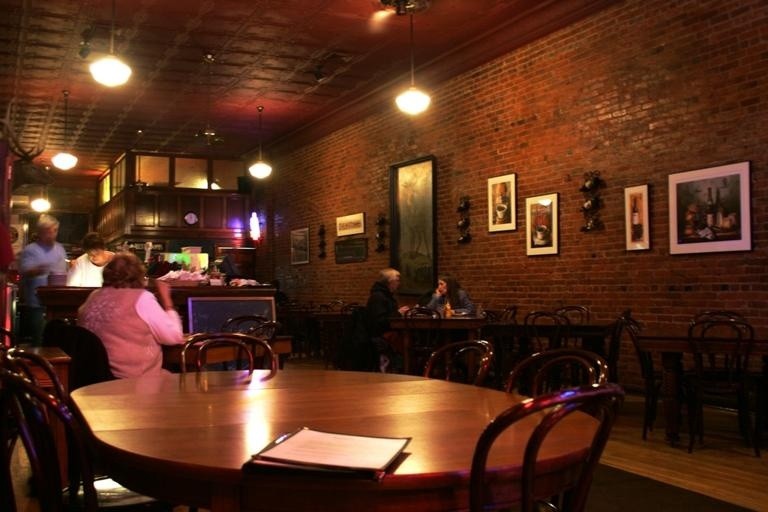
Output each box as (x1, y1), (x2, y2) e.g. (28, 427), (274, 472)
(704, 187), (714, 227)
(444, 302), (451, 317)
(633, 196), (639, 231)
(714, 188), (724, 227)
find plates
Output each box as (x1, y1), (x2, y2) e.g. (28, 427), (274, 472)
(533, 235), (549, 245)
(452, 313), (468, 317)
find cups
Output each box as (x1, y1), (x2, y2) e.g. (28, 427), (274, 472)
(496, 204), (507, 219)
(536, 224), (547, 240)
(728, 211), (737, 227)
(722, 217), (732, 229)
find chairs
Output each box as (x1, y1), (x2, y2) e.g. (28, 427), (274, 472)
(620, 309), (767, 458)
(1, 295), (629, 510)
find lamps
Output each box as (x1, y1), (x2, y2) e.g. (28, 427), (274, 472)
(84, 0), (133, 88)
(379, 0), (430, 117)
(49, 86), (80, 171)
(243, 102), (276, 181)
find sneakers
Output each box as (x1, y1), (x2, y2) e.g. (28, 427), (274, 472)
(379, 353), (391, 373)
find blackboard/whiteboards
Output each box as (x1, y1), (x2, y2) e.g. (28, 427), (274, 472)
(187, 296), (276, 335)
(28, 211), (90, 245)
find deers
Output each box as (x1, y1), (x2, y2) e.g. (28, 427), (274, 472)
(0, 121), (55, 191)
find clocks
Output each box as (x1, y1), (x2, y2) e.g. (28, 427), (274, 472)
(182, 210), (199, 226)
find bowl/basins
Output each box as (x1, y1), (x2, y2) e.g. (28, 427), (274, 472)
(209, 279), (221, 286)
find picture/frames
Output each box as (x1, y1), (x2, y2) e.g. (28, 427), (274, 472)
(668, 159), (755, 256)
(623, 184), (651, 252)
(486, 174), (518, 233)
(387, 153), (438, 299)
(289, 225), (312, 266)
(524, 192), (561, 257)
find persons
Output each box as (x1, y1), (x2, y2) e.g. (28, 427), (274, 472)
(75, 252), (184, 380)
(65, 232), (116, 287)
(17, 214), (69, 348)
(421, 274), (473, 316)
(361, 269), (411, 373)
